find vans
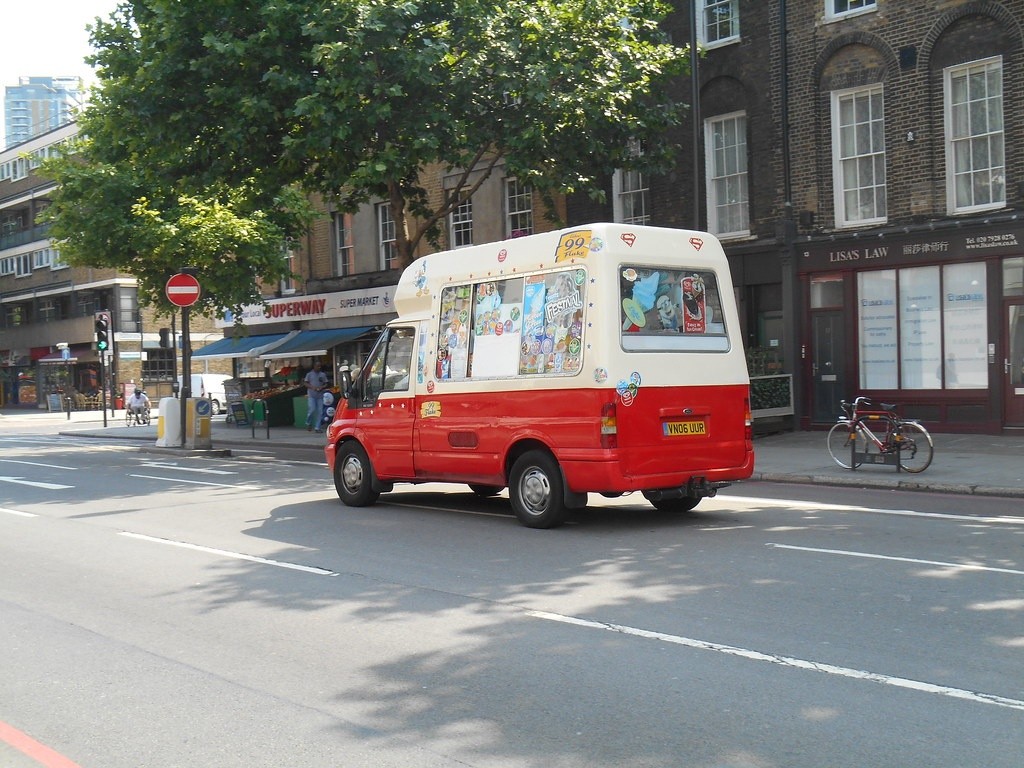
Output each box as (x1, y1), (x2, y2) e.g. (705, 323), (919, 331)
(173, 373), (234, 415)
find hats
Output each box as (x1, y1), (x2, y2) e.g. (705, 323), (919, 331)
(134, 388), (141, 393)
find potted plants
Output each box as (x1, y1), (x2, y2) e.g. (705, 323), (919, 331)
(113, 391), (124, 409)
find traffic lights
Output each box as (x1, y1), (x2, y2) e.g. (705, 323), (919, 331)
(96, 319), (108, 351)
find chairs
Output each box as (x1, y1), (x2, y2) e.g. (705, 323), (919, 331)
(78, 393), (91, 410)
(75, 393), (81, 410)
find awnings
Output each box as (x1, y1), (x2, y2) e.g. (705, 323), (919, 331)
(258, 326), (374, 359)
(37, 345), (100, 365)
(177, 332), (292, 361)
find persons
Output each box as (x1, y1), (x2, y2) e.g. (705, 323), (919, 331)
(127, 387), (151, 424)
(304, 361), (330, 433)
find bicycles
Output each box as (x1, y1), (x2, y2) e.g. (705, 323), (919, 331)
(827, 396), (934, 473)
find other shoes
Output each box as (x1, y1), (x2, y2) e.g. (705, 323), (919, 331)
(306, 427), (311, 431)
(316, 430), (322, 432)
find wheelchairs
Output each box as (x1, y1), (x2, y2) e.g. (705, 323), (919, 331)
(125, 391), (150, 427)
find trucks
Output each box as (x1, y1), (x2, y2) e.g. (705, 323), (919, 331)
(323, 221), (754, 527)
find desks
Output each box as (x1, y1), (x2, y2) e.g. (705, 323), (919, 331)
(293, 394), (318, 429)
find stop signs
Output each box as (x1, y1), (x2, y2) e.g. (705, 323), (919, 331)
(165, 274), (200, 307)
